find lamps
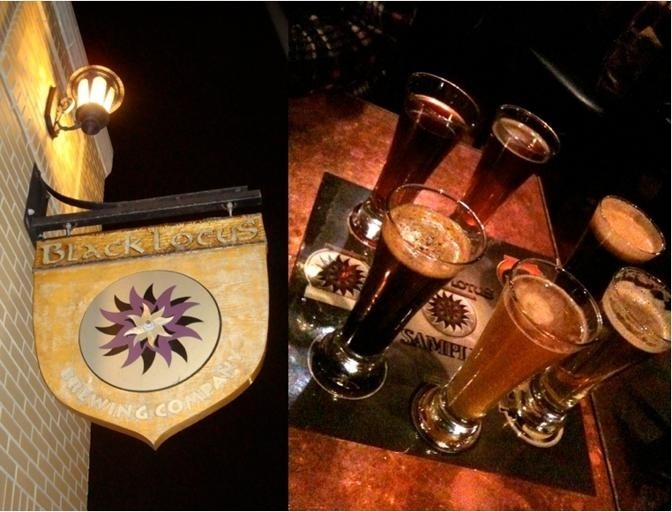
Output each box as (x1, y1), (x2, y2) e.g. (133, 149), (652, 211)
(44, 65), (125, 140)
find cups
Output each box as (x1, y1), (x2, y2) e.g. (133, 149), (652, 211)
(450, 104), (562, 236)
(347, 72), (482, 251)
(306, 183), (486, 401)
(409, 257), (602, 457)
(555, 194), (667, 307)
(498, 266), (670, 450)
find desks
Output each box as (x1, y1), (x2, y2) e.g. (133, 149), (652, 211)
(287, 82), (618, 510)
